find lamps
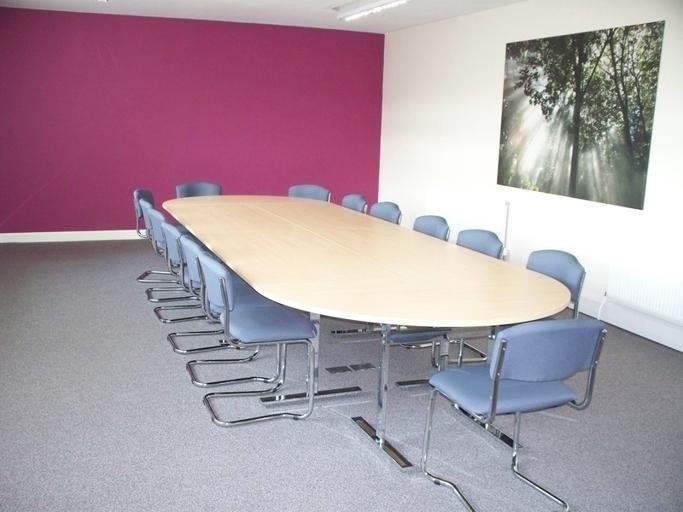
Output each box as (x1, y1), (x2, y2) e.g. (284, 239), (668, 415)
(332, 0), (408, 22)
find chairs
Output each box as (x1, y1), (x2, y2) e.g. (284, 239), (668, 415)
(176, 183), (220, 199)
(136, 199), (199, 304)
(144, 209), (210, 326)
(487, 250), (587, 342)
(369, 201), (403, 226)
(134, 185), (187, 283)
(162, 223), (243, 356)
(192, 249), (318, 429)
(454, 230), (504, 258)
(419, 316), (606, 512)
(180, 234), (283, 390)
(288, 183), (332, 202)
(342, 193), (369, 214)
(413, 215), (451, 241)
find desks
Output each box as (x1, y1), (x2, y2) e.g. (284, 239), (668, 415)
(161, 195), (571, 470)
